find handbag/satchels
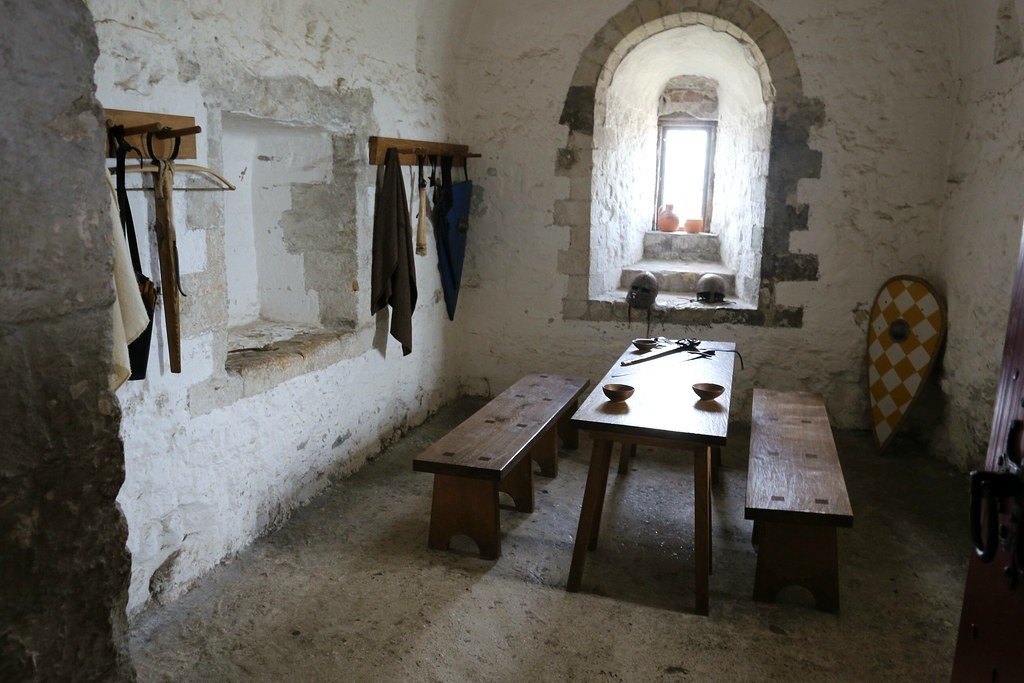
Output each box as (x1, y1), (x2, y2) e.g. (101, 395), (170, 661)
(127, 273), (153, 380)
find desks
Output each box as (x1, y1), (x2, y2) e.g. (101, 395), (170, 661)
(567, 337), (737, 616)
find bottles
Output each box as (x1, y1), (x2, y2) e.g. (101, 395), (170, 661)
(658, 204), (680, 232)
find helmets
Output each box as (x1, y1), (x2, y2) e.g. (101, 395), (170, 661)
(696, 273), (727, 301)
(626, 271), (659, 310)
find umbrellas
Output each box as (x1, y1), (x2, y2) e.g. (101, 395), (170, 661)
(432, 153), (472, 320)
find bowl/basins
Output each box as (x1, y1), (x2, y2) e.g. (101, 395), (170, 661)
(631, 338), (657, 352)
(603, 384), (635, 403)
(684, 219), (703, 233)
(692, 382), (725, 401)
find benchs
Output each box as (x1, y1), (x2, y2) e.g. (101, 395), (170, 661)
(412, 372), (591, 561)
(744, 387), (853, 614)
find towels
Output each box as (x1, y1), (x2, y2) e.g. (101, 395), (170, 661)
(104, 164), (151, 396)
(370, 146), (418, 356)
